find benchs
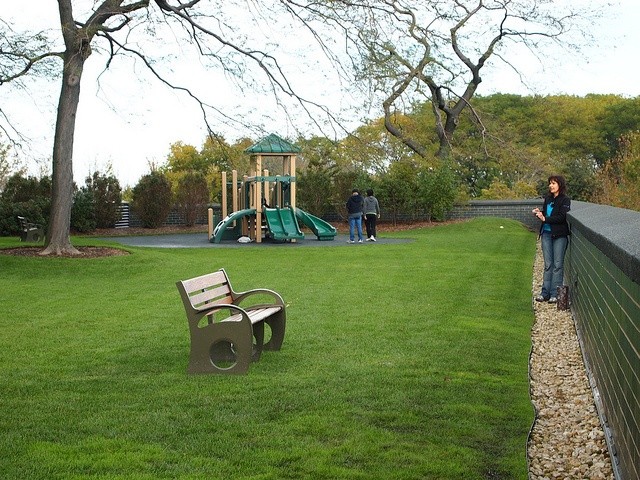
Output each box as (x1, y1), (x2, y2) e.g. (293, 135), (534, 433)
(17, 215), (44, 243)
(177, 269), (286, 378)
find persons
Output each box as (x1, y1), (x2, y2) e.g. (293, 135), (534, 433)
(347, 189), (364, 244)
(363, 189), (380, 242)
(531, 175), (571, 303)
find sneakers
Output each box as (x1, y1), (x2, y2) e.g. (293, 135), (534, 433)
(548, 296), (557, 303)
(370, 235), (376, 241)
(358, 239), (363, 243)
(346, 240), (355, 244)
(366, 238), (371, 241)
(536, 295), (544, 302)
